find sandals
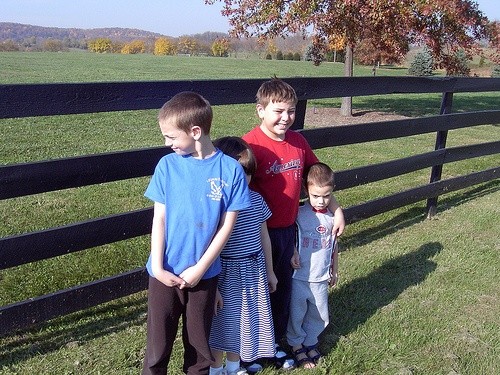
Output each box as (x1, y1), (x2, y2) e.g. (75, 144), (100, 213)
(307, 344), (322, 365)
(292, 344), (312, 365)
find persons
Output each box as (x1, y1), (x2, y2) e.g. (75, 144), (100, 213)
(241, 79), (345, 373)
(285, 163), (338, 369)
(142, 91), (251, 375)
(209, 136), (278, 375)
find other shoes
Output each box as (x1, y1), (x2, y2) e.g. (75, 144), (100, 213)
(271, 345), (297, 370)
(245, 360), (264, 371)
(227, 366), (246, 375)
